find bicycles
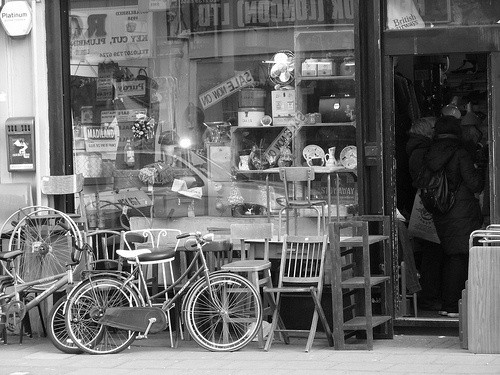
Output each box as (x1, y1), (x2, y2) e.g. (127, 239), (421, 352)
(62, 231), (263, 355)
(0, 204), (181, 354)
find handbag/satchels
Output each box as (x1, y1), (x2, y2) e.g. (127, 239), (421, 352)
(407, 186), (441, 244)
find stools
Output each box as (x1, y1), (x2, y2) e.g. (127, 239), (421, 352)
(122, 228), (182, 350)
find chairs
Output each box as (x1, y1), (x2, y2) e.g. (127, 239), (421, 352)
(220, 223), (276, 349)
(262, 234), (333, 353)
(279, 166), (327, 238)
(173, 239), (233, 349)
(0, 172), (127, 345)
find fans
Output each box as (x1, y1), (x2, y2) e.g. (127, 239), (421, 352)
(268, 50), (295, 90)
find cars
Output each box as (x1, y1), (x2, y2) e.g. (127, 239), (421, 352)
(74, 142), (325, 221)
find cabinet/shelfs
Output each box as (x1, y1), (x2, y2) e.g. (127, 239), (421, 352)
(292, 29), (357, 201)
(328, 214), (394, 350)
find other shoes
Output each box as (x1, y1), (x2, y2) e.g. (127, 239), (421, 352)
(447, 306), (460, 318)
(440, 304), (448, 317)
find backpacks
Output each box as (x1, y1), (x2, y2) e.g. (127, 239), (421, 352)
(419, 156), (461, 216)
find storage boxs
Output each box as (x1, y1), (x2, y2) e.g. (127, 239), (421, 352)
(317, 59), (336, 76)
(301, 58), (318, 77)
(237, 108), (265, 126)
(238, 88), (266, 108)
(272, 90), (295, 125)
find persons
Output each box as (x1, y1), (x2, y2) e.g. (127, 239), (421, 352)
(414, 115), (487, 318)
(406, 117), (435, 214)
